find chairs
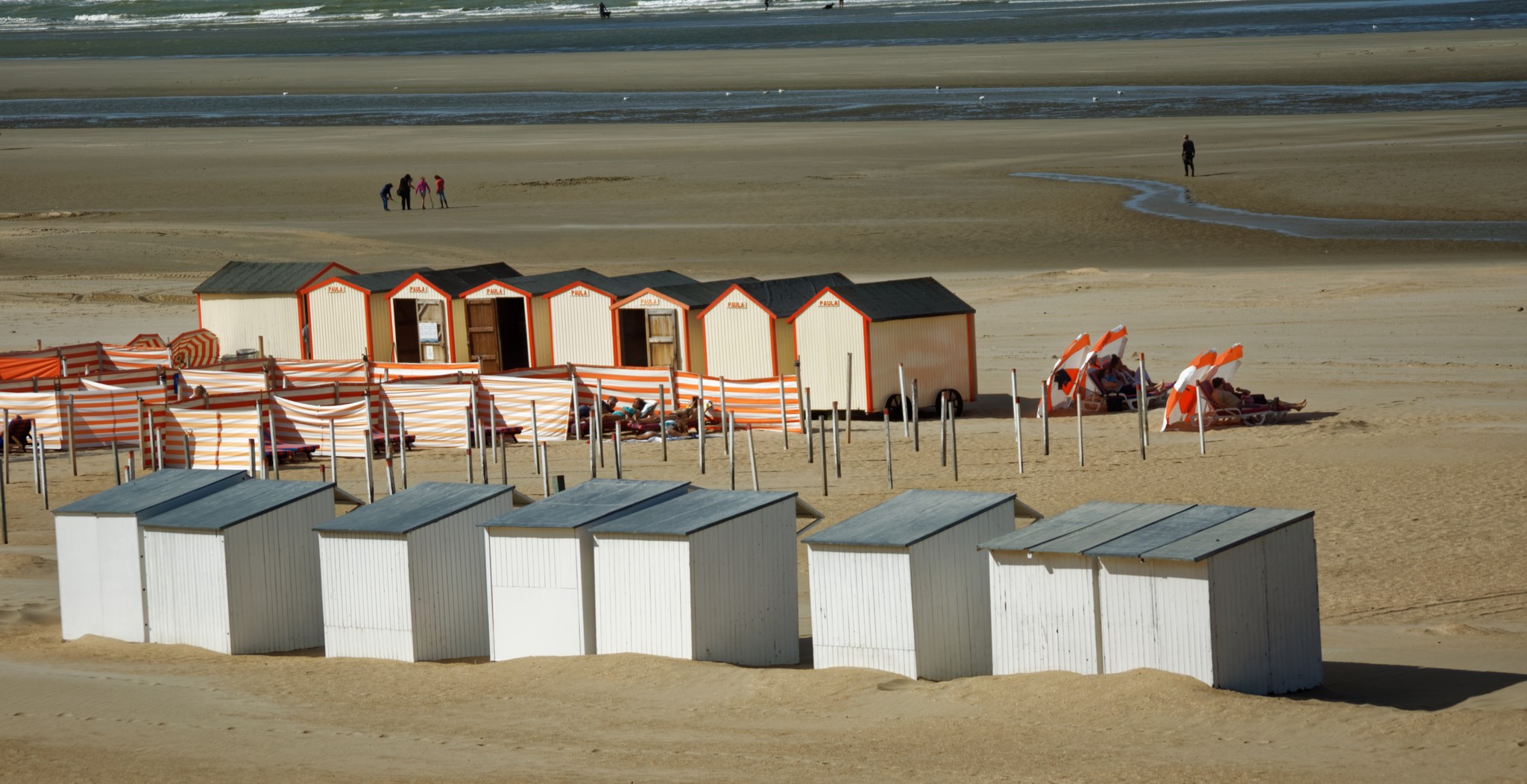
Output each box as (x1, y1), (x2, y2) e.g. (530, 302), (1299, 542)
(1081, 367), (1165, 412)
(627, 400), (714, 436)
(15, 418), (40, 453)
(469, 412), (523, 444)
(265, 449), (294, 464)
(373, 436), (395, 454)
(0, 415), (24, 453)
(263, 426), (320, 462)
(1188, 379), (1279, 428)
(372, 424), (416, 451)
(1221, 377), (1291, 422)
(470, 427), (491, 446)
(1097, 354), (1177, 399)
(580, 400), (658, 439)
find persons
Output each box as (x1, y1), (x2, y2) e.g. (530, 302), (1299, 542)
(435, 175), (449, 209)
(1182, 134), (1197, 179)
(1211, 376), (1308, 414)
(397, 174), (416, 210)
(1098, 353), (1165, 393)
(415, 177), (432, 210)
(380, 184), (394, 211)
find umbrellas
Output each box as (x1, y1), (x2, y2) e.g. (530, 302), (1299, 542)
(127, 333), (166, 349)
(1161, 347), (1217, 432)
(1200, 343), (1243, 417)
(1072, 325), (1128, 402)
(166, 329), (220, 369)
(1035, 332), (1109, 418)
